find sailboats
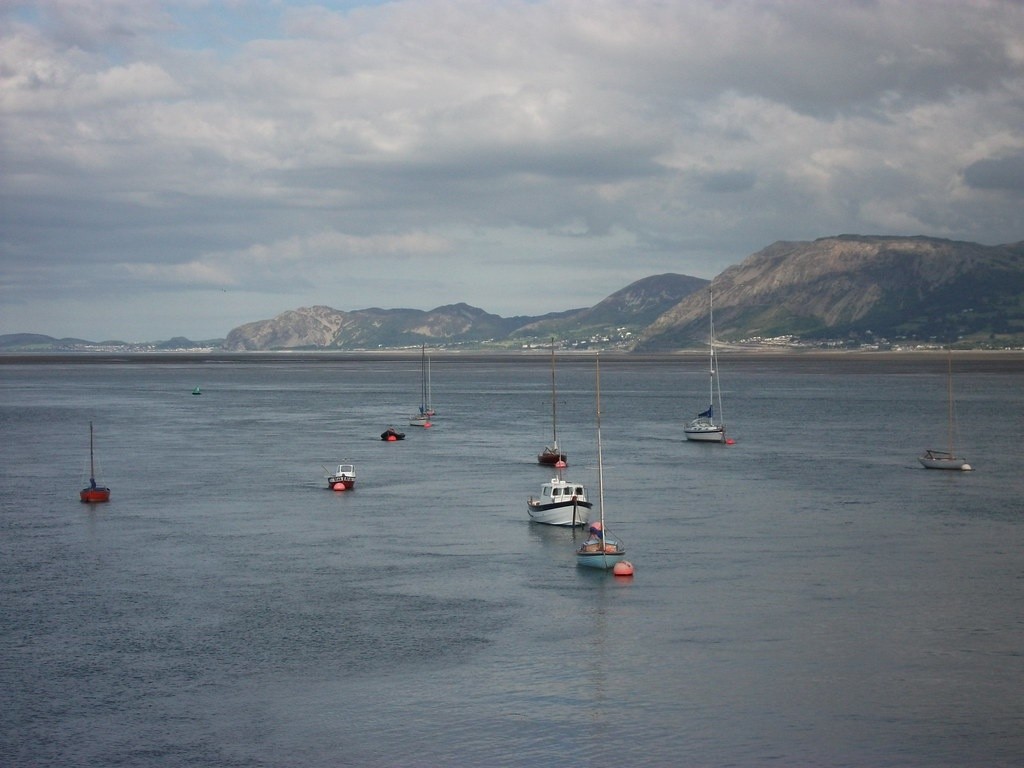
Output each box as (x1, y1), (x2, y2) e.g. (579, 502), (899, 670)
(526, 337), (594, 525)
(916, 342), (969, 470)
(192, 384), (202, 395)
(574, 352), (626, 567)
(683, 290), (728, 444)
(77, 420), (111, 503)
(408, 340), (430, 428)
(419, 354), (436, 416)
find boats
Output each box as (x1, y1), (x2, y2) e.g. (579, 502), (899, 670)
(381, 429), (405, 442)
(321, 464), (356, 491)
